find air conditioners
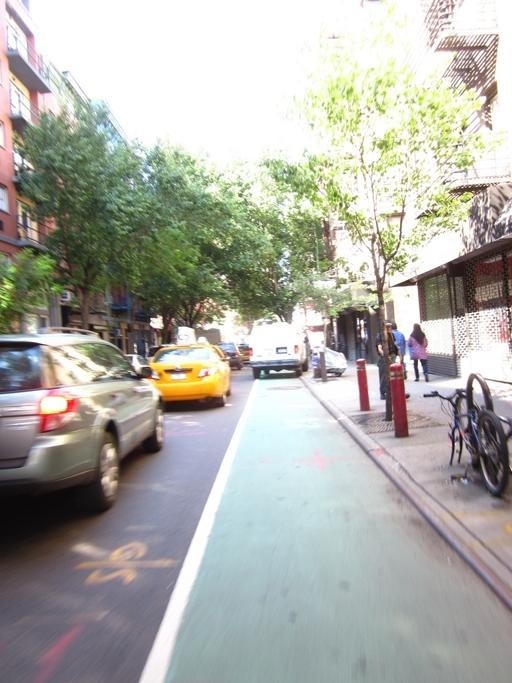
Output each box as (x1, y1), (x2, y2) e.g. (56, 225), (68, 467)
(61, 291), (74, 302)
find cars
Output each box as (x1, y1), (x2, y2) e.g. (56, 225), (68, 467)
(125, 353), (149, 374)
(218, 341), (245, 370)
(148, 343), (234, 408)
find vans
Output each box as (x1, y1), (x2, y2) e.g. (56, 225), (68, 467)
(247, 322), (310, 377)
(0, 326), (167, 517)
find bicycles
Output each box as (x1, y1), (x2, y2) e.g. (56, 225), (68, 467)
(424, 372), (512, 497)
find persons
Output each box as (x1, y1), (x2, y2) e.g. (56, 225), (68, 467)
(390, 322), (407, 380)
(407, 323), (430, 383)
(375, 322), (410, 399)
